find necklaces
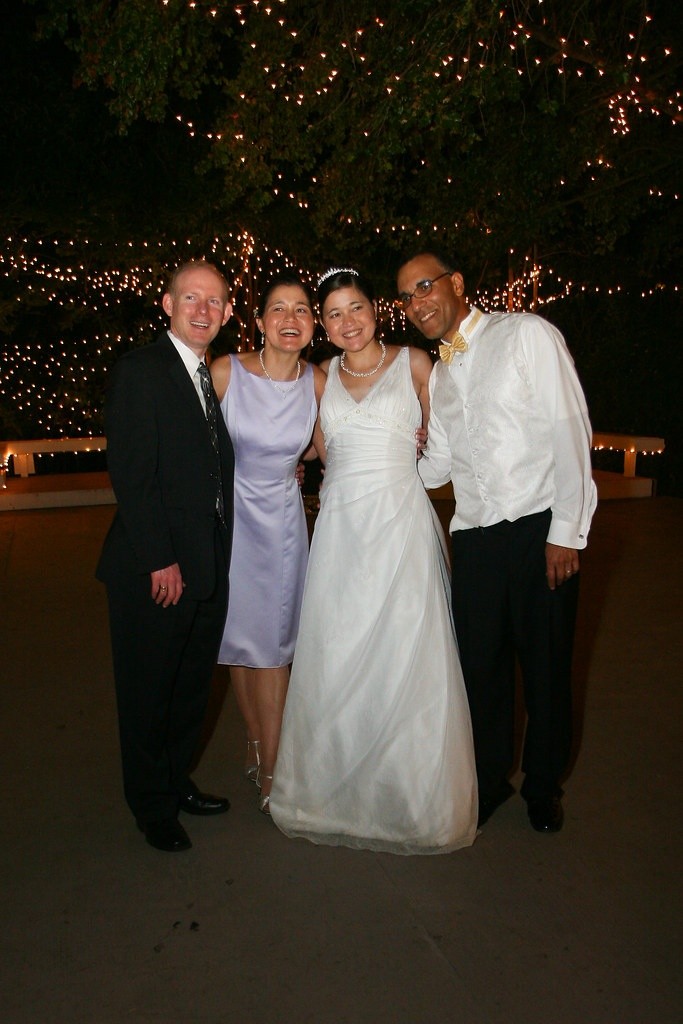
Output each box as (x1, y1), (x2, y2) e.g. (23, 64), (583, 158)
(259, 347), (301, 380)
(340, 341), (387, 379)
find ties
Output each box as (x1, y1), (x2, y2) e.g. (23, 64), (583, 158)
(197, 361), (228, 532)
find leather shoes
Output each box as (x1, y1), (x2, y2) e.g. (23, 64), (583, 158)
(178, 784), (231, 816)
(478, 780), (514, 828)
(134, 817), (192, 851)
(519, 769), (564, 831)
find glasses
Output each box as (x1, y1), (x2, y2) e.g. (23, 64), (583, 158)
(393, 273), (453, 310)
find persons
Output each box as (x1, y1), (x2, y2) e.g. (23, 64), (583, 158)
(268, 266), (480, 858)
(313, 252), (601, 834)
(207, 274), (428, 816)
(97, 260), (305, 853)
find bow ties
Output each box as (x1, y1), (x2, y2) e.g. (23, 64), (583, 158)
(439, 309), (482, 365)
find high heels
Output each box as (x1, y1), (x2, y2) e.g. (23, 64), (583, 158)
(255, 761), (273, 815)
(245, 738), (260, 781)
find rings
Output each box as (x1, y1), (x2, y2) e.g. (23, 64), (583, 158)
(565, 568), (572, 574)
(161, 586), (167, 592)
